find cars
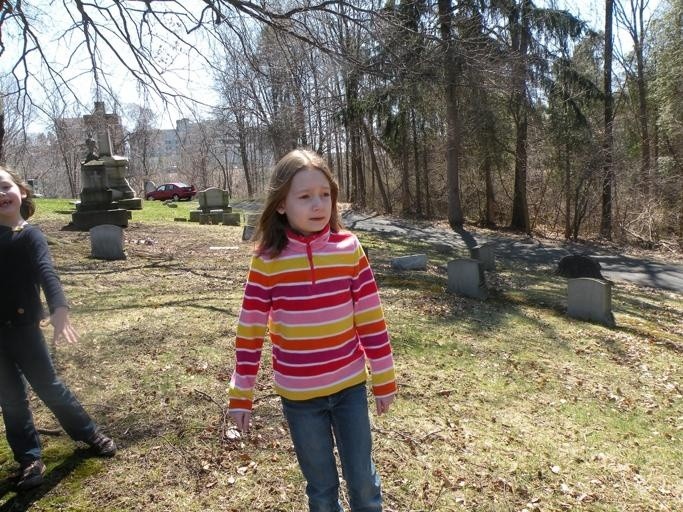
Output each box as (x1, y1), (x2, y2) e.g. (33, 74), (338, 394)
(144, 182), (197, 201)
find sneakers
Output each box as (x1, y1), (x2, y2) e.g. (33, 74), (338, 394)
(21, 458), (46, 486)
(84, 431), (115, 456)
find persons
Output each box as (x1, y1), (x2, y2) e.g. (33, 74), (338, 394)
(1, 163), (118, 492)
(225, 147), (398, 512)
